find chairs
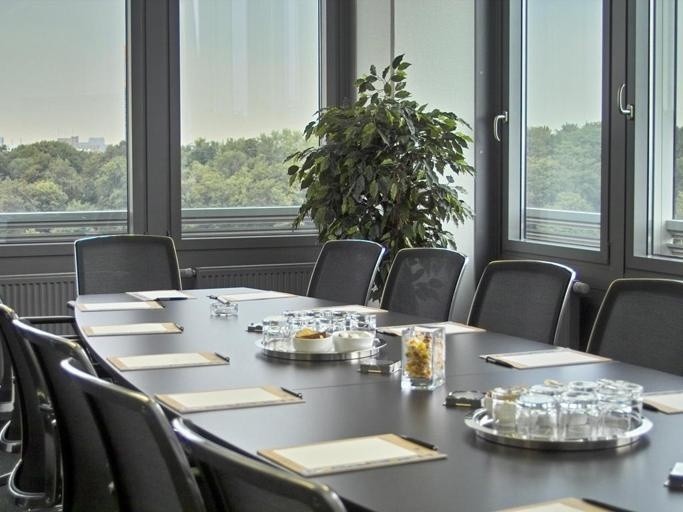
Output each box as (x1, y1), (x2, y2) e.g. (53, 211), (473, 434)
(58, 354), (208, 511)
(377, 246), (470, 323)
(581, 274), (681, 380)
(465, 257), (578, 347)
(2, 303), (84, 400)
(70, 236), (184, 296)
(10, 319), (111, 508)
(304, 237), (387, 306)
(170, 415), (348, 512)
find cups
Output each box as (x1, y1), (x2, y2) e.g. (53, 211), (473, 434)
(262, 309), (378, 344)
(487, 378), (643, 438)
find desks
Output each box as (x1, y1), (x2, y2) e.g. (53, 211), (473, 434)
(70, 283), (617, 512)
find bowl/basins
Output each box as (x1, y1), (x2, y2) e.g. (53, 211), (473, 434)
(294, 332), (374, 353)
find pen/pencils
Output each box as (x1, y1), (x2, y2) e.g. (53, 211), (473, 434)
(383, 332), (398, 336)
(216, 352), (230, 362)
(175, 323), (184, 331)
(209, 295), (218, 299)
(443, 402), (473, 407)
(156, 298), (166, 308)
(583, 497), (635, 512)
(357, 368), (381, 373)
(169, 297), (188, 300)
(486, 356), (513, 368)
(401, 434), (439, 450)
(281, 387), (303, 398)
(643, 403), (658, 411)
